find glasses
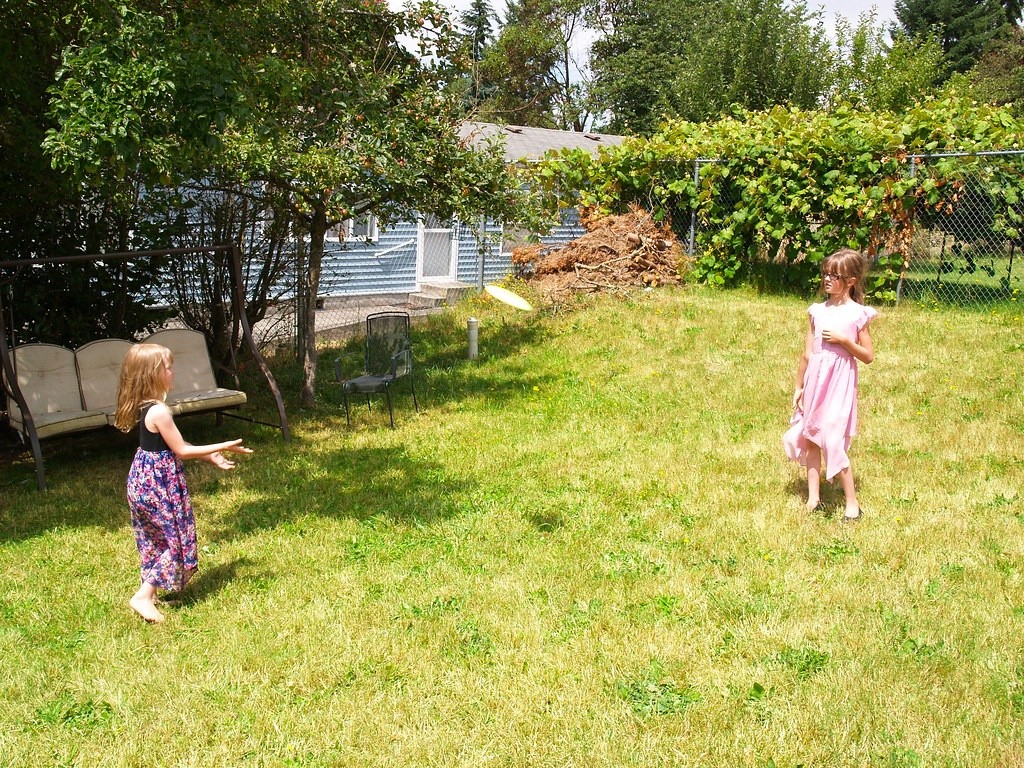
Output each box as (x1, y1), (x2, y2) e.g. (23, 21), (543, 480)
(820, 270), (848, 281)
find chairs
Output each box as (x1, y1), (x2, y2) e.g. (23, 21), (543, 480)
(3, 328), (247, 444)
(342, 309), (419, 429)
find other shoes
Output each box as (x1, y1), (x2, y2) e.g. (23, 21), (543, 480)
(843, 506), (859, 519)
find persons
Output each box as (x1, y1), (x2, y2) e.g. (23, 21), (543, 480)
(112, 344), (254, 625)
(784, 249), (878, 524)
